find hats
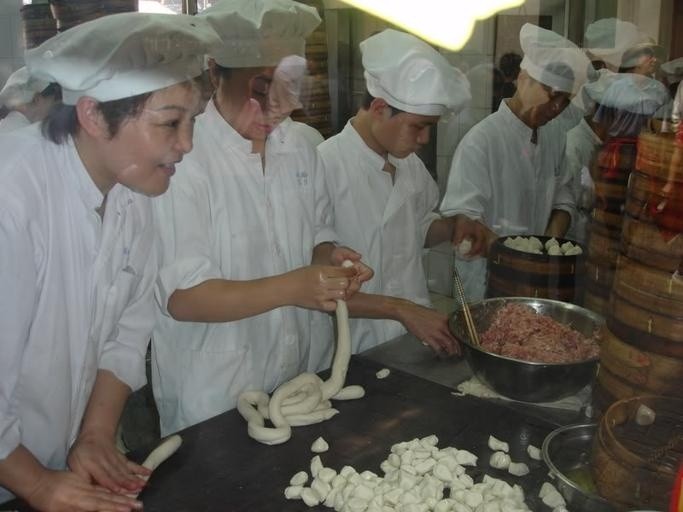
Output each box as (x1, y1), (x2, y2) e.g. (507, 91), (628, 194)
(357, 27), (474, 119)
(660, 56), (682, 87)
(584, 14), (655, 68)
(24, 12), (214, 104)
(516, 21), (591, 101)
(0, 65), (59, 110)
(194, 0), (321, 70)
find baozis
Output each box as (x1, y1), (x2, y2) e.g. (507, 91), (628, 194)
(503, 236), (583, 256)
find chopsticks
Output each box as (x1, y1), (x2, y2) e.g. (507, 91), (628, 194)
(448, 265), (487, 345)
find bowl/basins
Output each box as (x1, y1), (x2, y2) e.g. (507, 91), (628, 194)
(450, 295), (607, 403)
